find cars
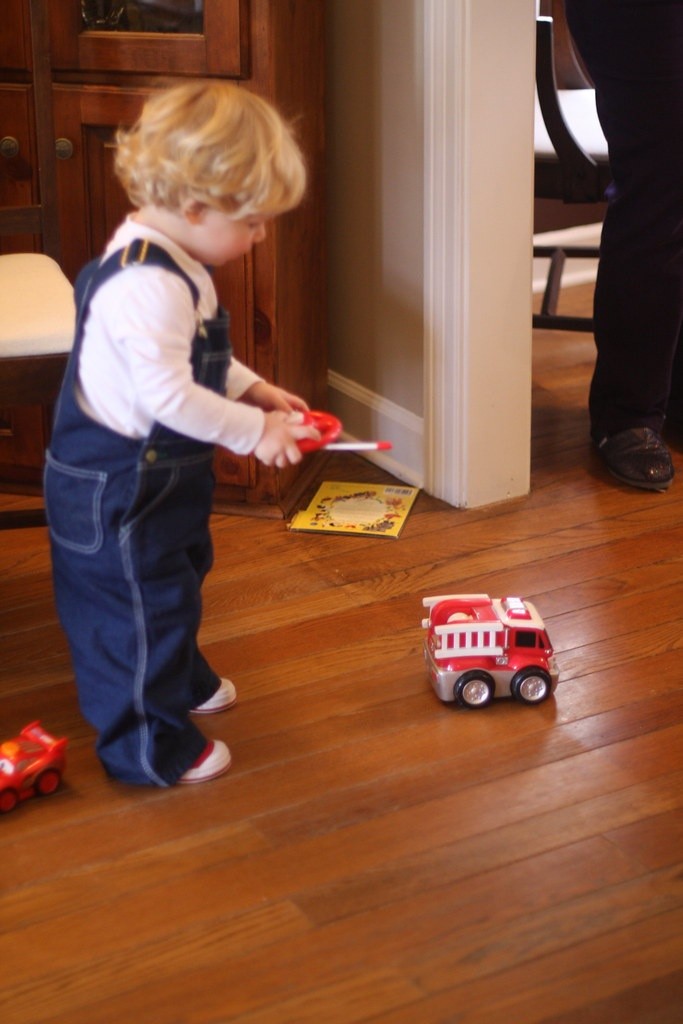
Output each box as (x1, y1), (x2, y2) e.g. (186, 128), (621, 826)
(0, 720), (72, 816)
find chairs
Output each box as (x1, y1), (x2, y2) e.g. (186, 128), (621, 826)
(527, 14), (614, 335)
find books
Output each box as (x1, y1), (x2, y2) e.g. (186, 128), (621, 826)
(290, 480), (419, 538)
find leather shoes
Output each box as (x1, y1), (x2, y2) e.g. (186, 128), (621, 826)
(593, 426), (674, 485)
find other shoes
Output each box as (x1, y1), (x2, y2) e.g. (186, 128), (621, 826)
(190, 678), (236, 715)
(181, 736), (231, 786)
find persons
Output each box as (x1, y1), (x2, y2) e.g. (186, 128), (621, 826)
(563, 1), (682, 492)
(44, 78), (320, 789)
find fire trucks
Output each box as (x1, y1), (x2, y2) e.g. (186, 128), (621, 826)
(420, 591), (563, 712)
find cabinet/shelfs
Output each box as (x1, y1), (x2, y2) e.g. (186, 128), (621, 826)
(0, 0), (332, 518)
